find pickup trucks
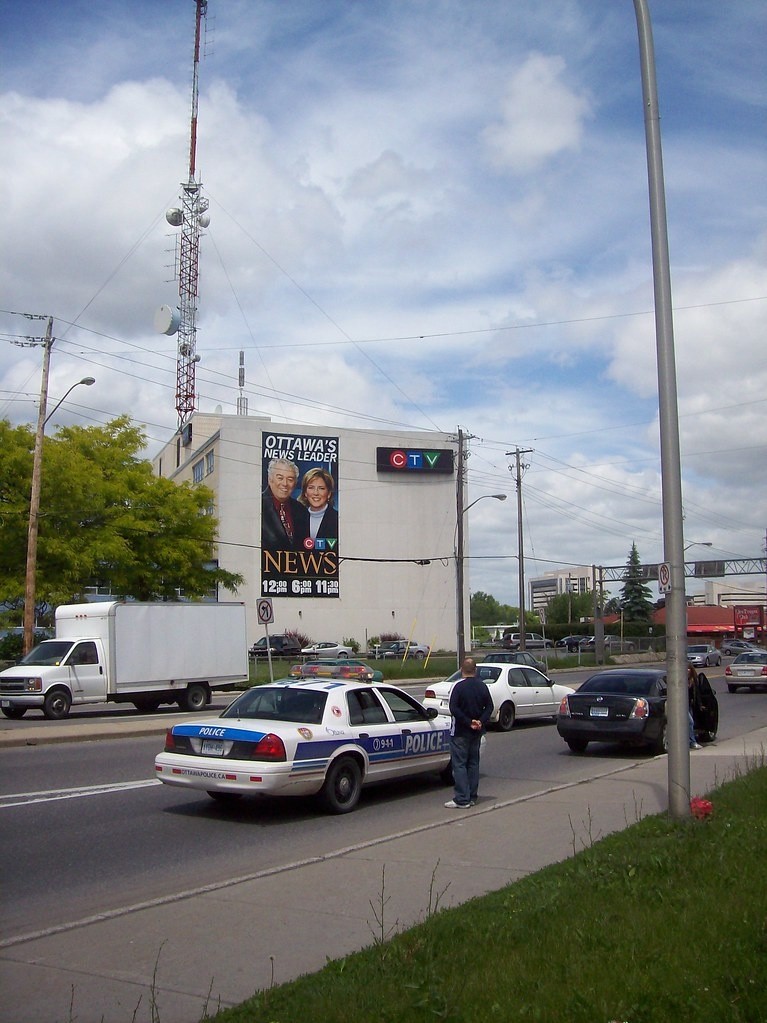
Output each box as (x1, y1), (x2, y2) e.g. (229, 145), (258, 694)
(390, 641), (430, 660)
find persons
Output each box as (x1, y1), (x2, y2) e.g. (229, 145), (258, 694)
(444, 657), (494, 808)
(685, 662), (707, 748)
(262, 458), (336, 577)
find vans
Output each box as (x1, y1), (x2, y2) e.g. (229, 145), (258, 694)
(503, 633), (553, 651)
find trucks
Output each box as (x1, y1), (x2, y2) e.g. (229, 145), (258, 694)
(0, 601), (250, 720)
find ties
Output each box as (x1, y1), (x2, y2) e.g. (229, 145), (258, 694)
(280, 504), (293, 544)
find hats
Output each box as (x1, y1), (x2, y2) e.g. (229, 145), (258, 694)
(462, 658), (476, 677)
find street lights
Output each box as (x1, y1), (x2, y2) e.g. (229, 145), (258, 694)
(456, 494), (507, 669)
(23, 377), (95, 658)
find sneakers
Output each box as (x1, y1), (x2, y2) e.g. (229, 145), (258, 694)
(691, 743), (703, 748)
(444, 800), (470, 808)
(470, 799), (480, 805)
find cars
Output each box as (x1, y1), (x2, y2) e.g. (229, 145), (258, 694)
(421, 662), (577, 732)
(154, 664), (485, 814)
(555, 635), (587, 652)
(721, 641), (767, 656)
(301, 642), (355, 659)
(724, 652), (767, 694)
(367, 640), (410, 660)
(588, 635), (635, 652)
(578, 636), (595, 650)
(687, 644), (723, 667)
(556, 669), (719, 756)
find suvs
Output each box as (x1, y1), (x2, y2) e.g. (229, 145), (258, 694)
(248, 633), (302, 661)
